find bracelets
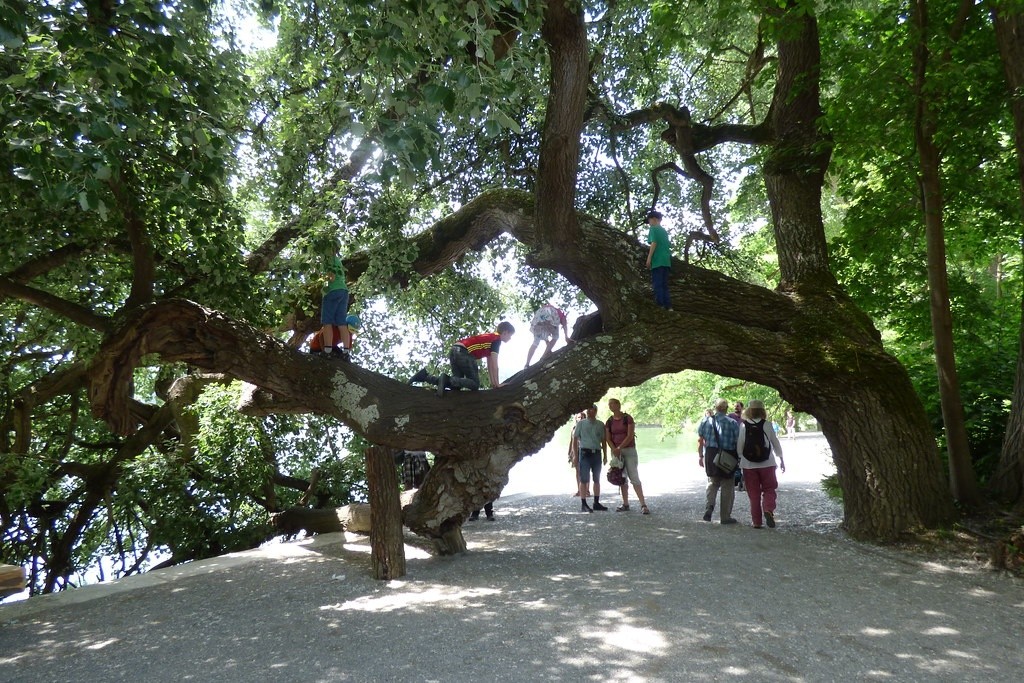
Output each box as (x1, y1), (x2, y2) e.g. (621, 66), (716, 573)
(618, 447), (621, 449)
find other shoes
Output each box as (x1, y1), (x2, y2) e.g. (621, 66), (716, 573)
(593, 503), (608, 510)
(488, 515), (495, 521)
(721, 518), (736, 524)
(703, 509), (713, 521)
(764, 510), (775, 528)
(337, 352), (350, 361)
(751, 521), (761, 527)
(469, 516), (478, 521)
(317, 350), (333, 359)
(582, 505), (593, 512)
(438, 372), (451, 397)
(586, 491), (590, 496)
(407, 367), (428, 385)
(573, 490), (580, 497)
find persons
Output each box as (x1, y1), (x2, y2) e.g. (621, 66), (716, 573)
(524, 305), (574, 368)
(643, 211), (673, 311)
(697, 398), (740, 524)
(300, 467), (332, 537)
(786, 411), (795, 441)
(568, 412), (590, 497)
(605, 399), (649, 514)
(408, 322), (515, 397)
(401, 451), (430, 490)
(772, 419), (779, 437)
(737, 400), (785, 529)
(727, 402), (744, 425)
(468, 502), (495, 521)
(310, 315), (360, 355)
(572, 404), (608, 513)
(705, 408), (713, 416)
(320, 237), (350, 360)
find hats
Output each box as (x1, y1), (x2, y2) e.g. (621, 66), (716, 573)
(346, 315), (362, 335)
(750, 400), (764, 408)
(643, 211), (661, 224)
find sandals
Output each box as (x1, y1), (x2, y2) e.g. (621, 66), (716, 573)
(641, 505), (650, 515)
(615, 504), (630, 511)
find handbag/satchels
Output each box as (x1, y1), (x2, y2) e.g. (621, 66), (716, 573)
(713, 449), (739, 474)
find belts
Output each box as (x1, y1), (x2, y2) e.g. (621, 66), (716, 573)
(581, 448), (600, 453)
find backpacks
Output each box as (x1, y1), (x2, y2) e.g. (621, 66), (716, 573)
(743, 419), (771, 462)
